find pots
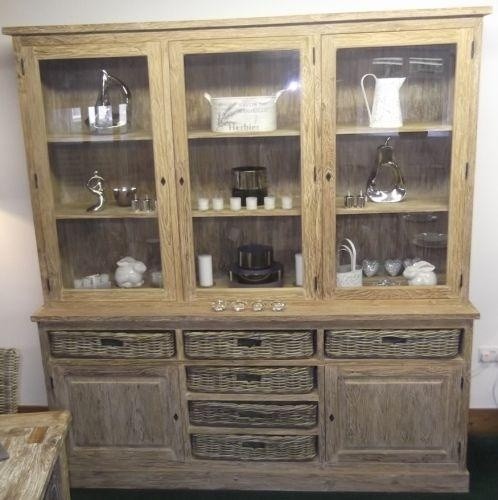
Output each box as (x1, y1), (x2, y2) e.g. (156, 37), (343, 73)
(201, 88), (285, 132)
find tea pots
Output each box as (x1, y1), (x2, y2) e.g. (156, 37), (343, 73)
(359, 72), (407, 128)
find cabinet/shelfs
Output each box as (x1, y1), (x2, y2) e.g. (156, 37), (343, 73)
(324, 321), (474, 498)
(35, 324), (186, 498)
(320, 18), (482, 302)
(11, 20), (320, 305)
(177, 324), (323, 497)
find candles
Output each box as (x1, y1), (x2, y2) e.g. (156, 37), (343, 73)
(197, 251), (215, 290)
(293, 251), (304, 288)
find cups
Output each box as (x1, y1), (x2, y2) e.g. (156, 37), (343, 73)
(112, 187), (137, 207)
(151, 271), (162, 288)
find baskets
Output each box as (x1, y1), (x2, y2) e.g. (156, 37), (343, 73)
(182, 330), (314, 361)
(191, 433), (318, 462)
(48, 330), (175, 359)
(184, 365), (315, 394)
(188, 400), (318, 429)
(324, 327), (462, 360)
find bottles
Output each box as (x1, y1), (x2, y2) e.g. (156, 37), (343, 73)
(365, 135), (408, 204)
(344, 188), (365, 207)
(132, 192), (154, 212)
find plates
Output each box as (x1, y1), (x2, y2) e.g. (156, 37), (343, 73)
(411, 232), (448, 250)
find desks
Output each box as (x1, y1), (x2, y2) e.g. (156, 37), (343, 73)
(0, 408), (74, 500)
